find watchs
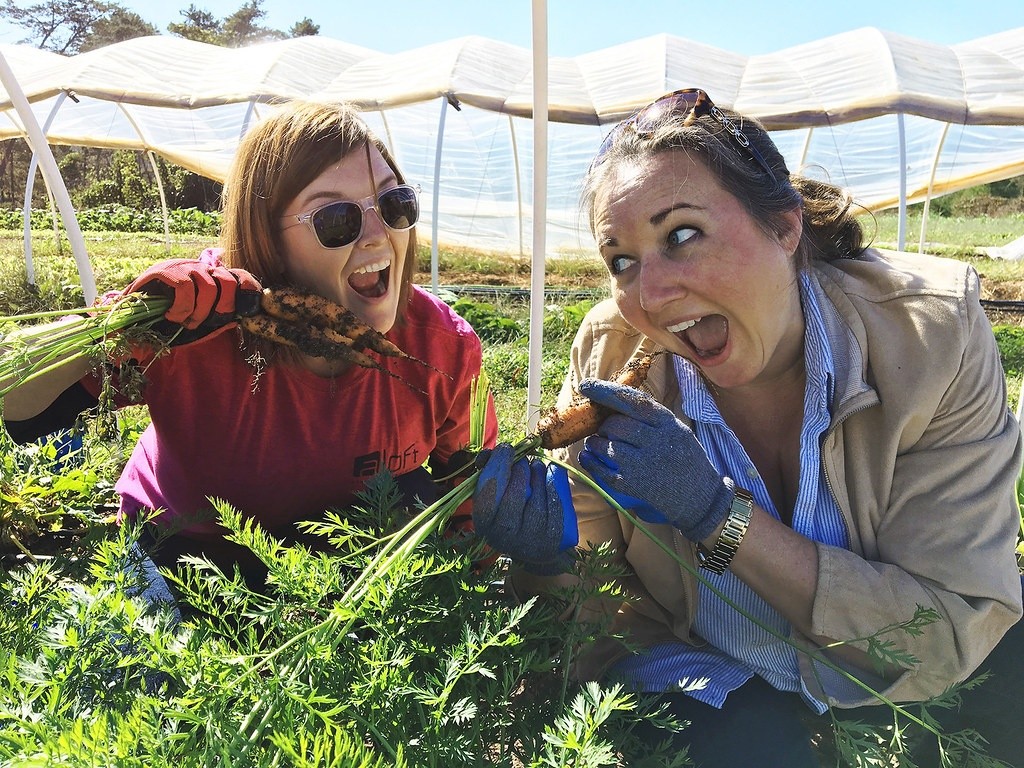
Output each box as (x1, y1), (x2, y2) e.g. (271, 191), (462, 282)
(695, 487), (756, 573)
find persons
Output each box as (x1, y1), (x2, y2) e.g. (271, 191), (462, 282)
(0, 103), (498, 653)
(473, 89), (1024, 768)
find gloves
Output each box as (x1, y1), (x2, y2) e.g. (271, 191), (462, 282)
(578, 377), (736, 544)
(446, 444), (579, 575)
(78, 248), (263, 375)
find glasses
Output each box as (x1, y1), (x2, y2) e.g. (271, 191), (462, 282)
(277, 183), (422, 249)
(598, 88), (780, 187)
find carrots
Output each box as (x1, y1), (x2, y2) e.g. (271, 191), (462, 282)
(535, 346), (719, 450)
(239, 286), (453, 398)
